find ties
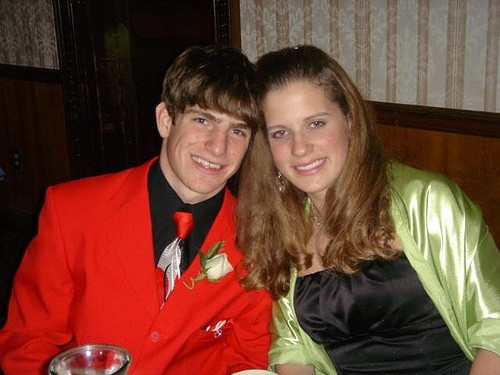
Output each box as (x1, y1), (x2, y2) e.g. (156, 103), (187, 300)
(154, 212), (194, 305)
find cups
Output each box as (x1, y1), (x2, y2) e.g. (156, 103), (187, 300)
(48, 343), (131, 375)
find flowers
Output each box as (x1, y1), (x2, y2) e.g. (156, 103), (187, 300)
(182, 239), (234, 291)
